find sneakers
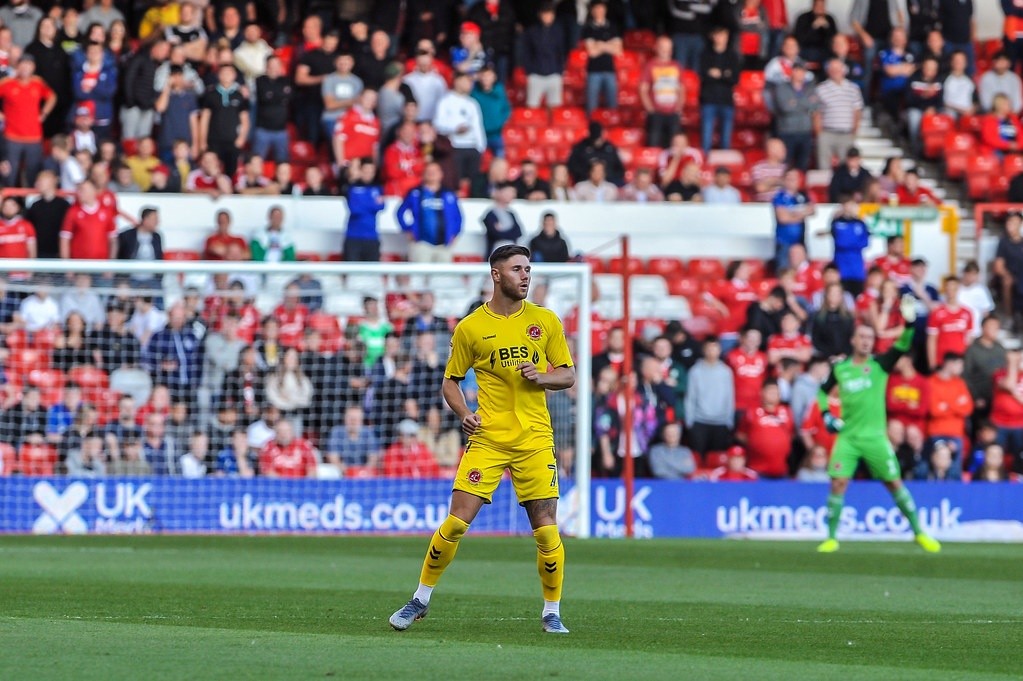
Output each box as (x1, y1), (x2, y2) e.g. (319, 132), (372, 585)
(543, 613), (570, 633)
(390, 598), (429, 631)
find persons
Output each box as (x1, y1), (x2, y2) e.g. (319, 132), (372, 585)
(1, 0), (1023, 485)
(388, 244), (576, 634)
(811, 294), (941, 554)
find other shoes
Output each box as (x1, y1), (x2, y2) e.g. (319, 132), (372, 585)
(817, 538), (840, 553)
(916, 532), (941, 553)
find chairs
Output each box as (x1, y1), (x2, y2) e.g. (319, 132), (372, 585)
(564, 257), (783, 351)
(0, 329), (120, 423)
(500, 31), (1023, 203)
(304, 310), (351, 352)
(234, 121), (333, 190)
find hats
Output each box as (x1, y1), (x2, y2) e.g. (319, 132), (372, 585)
(728, 445), (745, 459)
(398, 418), (420, 435)
